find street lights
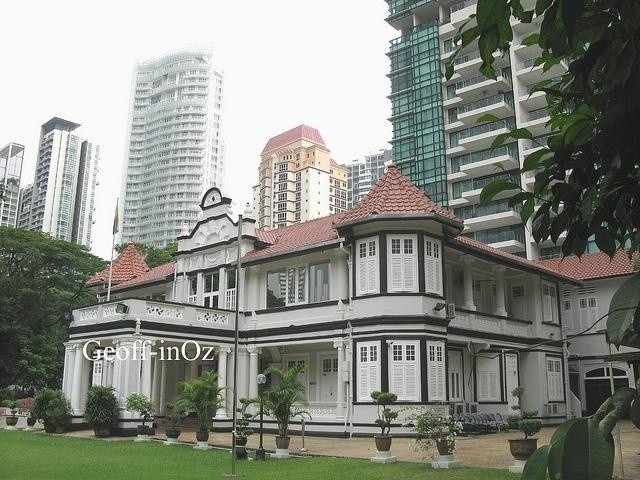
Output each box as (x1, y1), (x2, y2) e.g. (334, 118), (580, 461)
(256, 373), (267, 461)
(230, 200), (246, 475)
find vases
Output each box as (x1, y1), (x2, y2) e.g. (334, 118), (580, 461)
(435, 439), (456, 460)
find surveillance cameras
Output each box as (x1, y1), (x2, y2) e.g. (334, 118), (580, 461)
(386, 340), (393, 345)
(549, 331), (554, 336)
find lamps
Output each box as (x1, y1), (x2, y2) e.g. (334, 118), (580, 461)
(115, 303), (129, 315)
(433, 301), (446, 314)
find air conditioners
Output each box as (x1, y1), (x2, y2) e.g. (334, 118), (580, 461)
(447, 302), (458, 320)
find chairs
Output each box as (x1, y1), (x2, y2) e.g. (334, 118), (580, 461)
(453, 409), (514, 438)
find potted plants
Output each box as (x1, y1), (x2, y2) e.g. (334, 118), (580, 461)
(231, 397), (257, 446)
(505, 415), (545, 461)
(89, 384), (123, 438)
(169, 369), (235, 445)
(369, 386), (404, 456)
(29, 386), (78, 435)
(165, 413), (186, 440)
(246, 362), (316, 451)
(2, 396), (20, 427)
(25, 406), (37, 426)
(125, 391), (158, 435)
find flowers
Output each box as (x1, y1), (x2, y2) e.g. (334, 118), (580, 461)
(408, 407), (461, 459)
